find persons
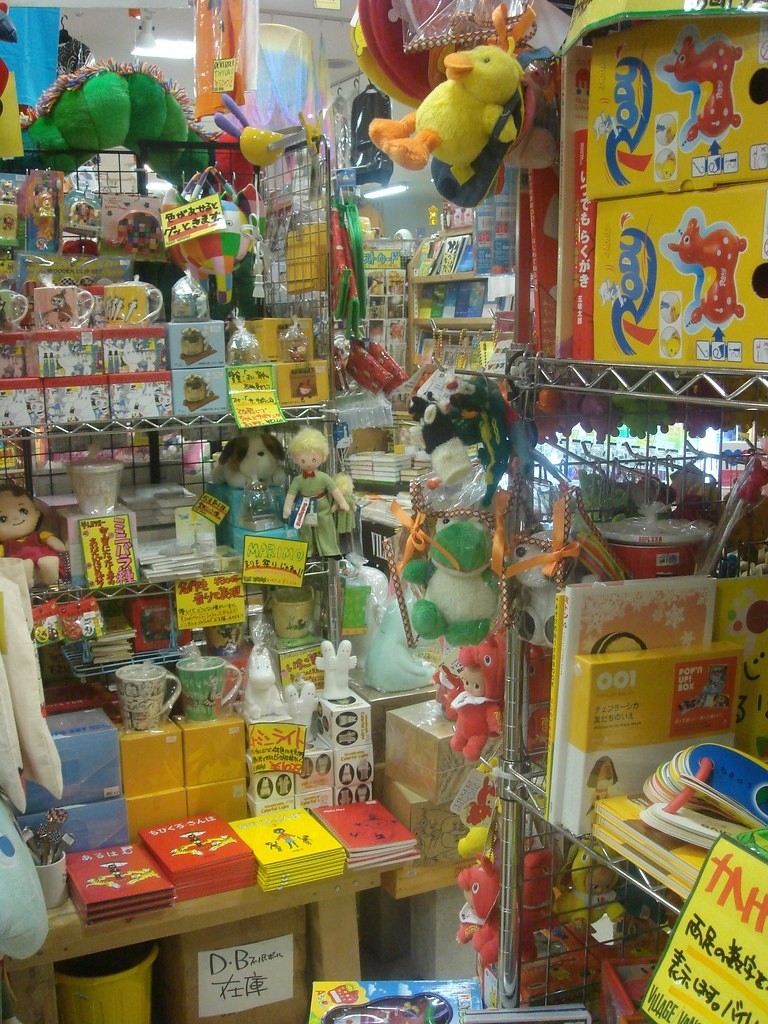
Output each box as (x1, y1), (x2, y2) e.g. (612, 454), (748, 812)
(0, 481), (68, 592)
(330, 472), (357, 535)
(281, 426), (350, 560)
(428, 640), (633, 969)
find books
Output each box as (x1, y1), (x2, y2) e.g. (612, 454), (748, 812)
(455, 573), (768, 1024)
(358, 235), (518, 372)
(88, 612), (139, 706)
(115, 480), (209, 583)
(65, 797), (424, 928)
(348, 410), (431, 532)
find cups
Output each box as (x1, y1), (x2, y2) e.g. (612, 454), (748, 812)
(69, 460), (124, 515)
(31, 849), (69, 909)
(33, 286), (95, 330)
(176, 657), (242, 722)
(0, 290), (29, 332)
(114, 664), (182, 730)
(174, 293), (197, 317)
(271, 591), (314, 640)
(103, 283), (163, 327)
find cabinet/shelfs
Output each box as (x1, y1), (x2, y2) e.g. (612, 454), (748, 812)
(0, 403), (342, 713)
(407, 225), (492, 379)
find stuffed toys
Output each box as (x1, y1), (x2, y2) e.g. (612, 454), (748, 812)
(394, 374), (767, 647)
(368, 45), (525, 174)
(0, 55), (255, 198)
(159, 164), (266, 305)
(210, 426), (285, 490)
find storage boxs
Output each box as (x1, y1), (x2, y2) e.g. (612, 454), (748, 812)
(0, 329), (104, 378)
(229, 318), (330, 406)
(116, 716), (185, 798)
(18, 797), (129, 854)
(382, 769), (469, 867)
(127, 787), (187, 845)
(108, 371), (174, 419)
(0, 377), (46, 428)
(13, 707), (122, 816)
(174, 710), (246, 787)
(246, 733), (374, 818)
(101, 326), (167, 374)
(348, 681), (478, 805)
(166, 320), (226, 369)
(186, 778), (247, 822)
(171, 367), (227, 416)
(314, 689), (373, 748)
(43, 375), (110, 424)
(481, 17), (768, 1024)
